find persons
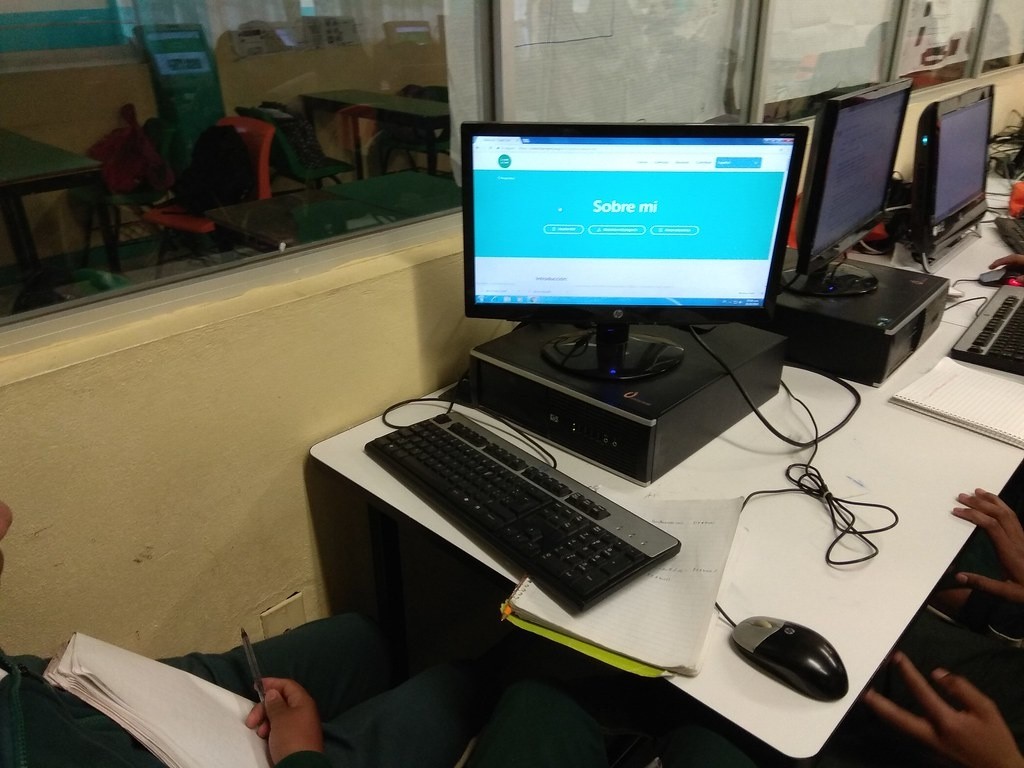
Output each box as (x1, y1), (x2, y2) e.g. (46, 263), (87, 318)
(0, 504), (492, 768)
(925, 254), (1024, 644)
(862, 488), (1024, 768)
(464, 681), (759, 768)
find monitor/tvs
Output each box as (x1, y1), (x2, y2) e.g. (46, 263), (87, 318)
(461, 119), (807, 381)
(911, 84), (995, 274)
(791, 78), (915, 296)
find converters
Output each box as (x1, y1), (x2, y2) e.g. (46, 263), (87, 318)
(994, 158), (1016, 179)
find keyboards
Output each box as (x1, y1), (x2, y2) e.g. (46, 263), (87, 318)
(363, 411), (682, 613)
(951, 285), (1024, 376)
(997, 218), (1024, 256)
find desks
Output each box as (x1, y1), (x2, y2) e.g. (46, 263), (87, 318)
(199, 169), (460, 250)
(302, 146), (1024, 758)
(0, 129), (122, 276)
(301, 88), (449, 187)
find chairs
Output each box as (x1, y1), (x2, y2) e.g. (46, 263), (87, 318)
(380, 82), (449, 175)
(81, 105), (357, 281)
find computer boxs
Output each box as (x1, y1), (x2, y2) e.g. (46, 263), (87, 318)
(758, 260), (950, 389)
(468, 320), (787, 488)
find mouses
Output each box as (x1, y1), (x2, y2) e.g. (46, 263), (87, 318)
(978, 267), (1022, 287)
(731, 616), (848, 703)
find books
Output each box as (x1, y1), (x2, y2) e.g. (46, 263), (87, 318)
(889, 356), (1024, 450)
(501, 483), (745, 677)
(43, 630), (276, 768)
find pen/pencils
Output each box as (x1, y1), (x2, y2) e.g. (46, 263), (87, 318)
(240, 628), (265, 703)
